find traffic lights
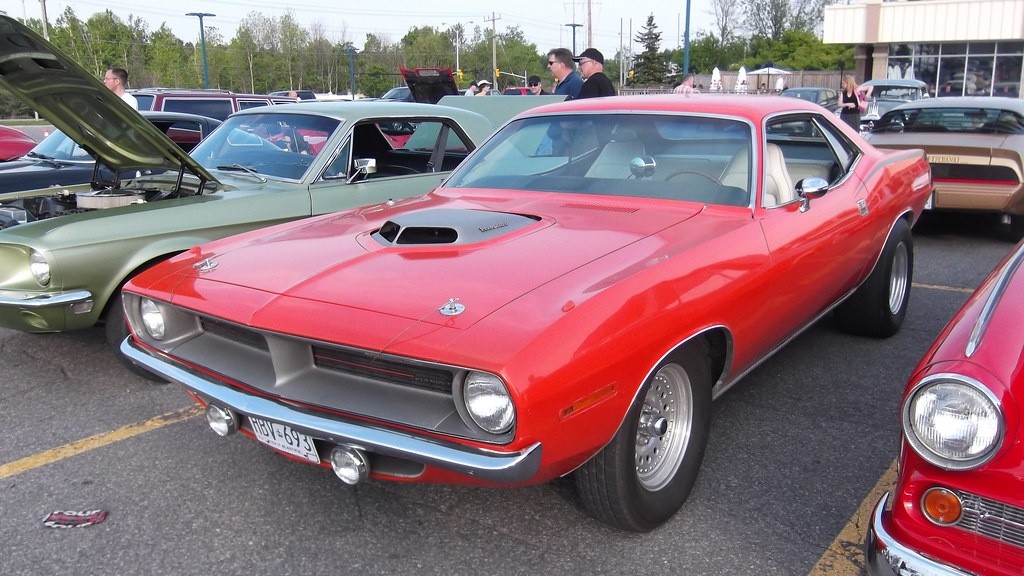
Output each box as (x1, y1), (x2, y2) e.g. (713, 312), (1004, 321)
(496, 68), (500, 77)
(459, 69), (464, 81)
(629, 70), (634, 78)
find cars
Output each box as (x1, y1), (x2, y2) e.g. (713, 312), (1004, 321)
(1, 12), (933, 535)
(761, 86), (839, 138)
(862, 236), (1024, 576)
(870, 95), (1024, 242)
(935, 73), (1020, 98)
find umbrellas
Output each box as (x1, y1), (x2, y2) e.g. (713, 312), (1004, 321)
(747, 68), (793, 88)
(734, 67), (747, 94)
(709, 67), (722, 91)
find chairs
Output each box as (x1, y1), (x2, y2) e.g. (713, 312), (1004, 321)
(584, 129), (652, 181)
(718, 143), (793, 207)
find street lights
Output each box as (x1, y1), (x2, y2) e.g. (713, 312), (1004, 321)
(441, 21), (474, 89)
(185, 11), (216, 90)
(342, 48), (360, 100)
(565, 23), (583, 56)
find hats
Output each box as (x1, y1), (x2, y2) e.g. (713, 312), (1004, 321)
(572, 48), (603, 66)
(477, 80), (493, 87)
(529, 76), (541, 83)
(470, 81), (478, 87)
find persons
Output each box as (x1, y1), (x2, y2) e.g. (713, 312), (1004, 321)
(103, 68), (138, 111)
(762, 82), (766, 93)
(284, 130), (307, 154)
(528, 76), (559, 95)
(559, 49), (616, 178)
(673, 73), (694, 94)
(548, 49), (583, 156)
(289, 91), (301, 101)
(465, 80), (492, 96)
(774, 76), (783, 91)
(922, 87), (929, 97)
(838, 75), (873, 133)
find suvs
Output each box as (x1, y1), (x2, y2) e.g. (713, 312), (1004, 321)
(854, 79), (932, 136)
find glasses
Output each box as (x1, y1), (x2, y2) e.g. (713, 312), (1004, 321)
(548, 60), (560, 66)
(529, 82), (542, 87)
(578, 60), (593, 66)
(103, 77), (119, 84)
